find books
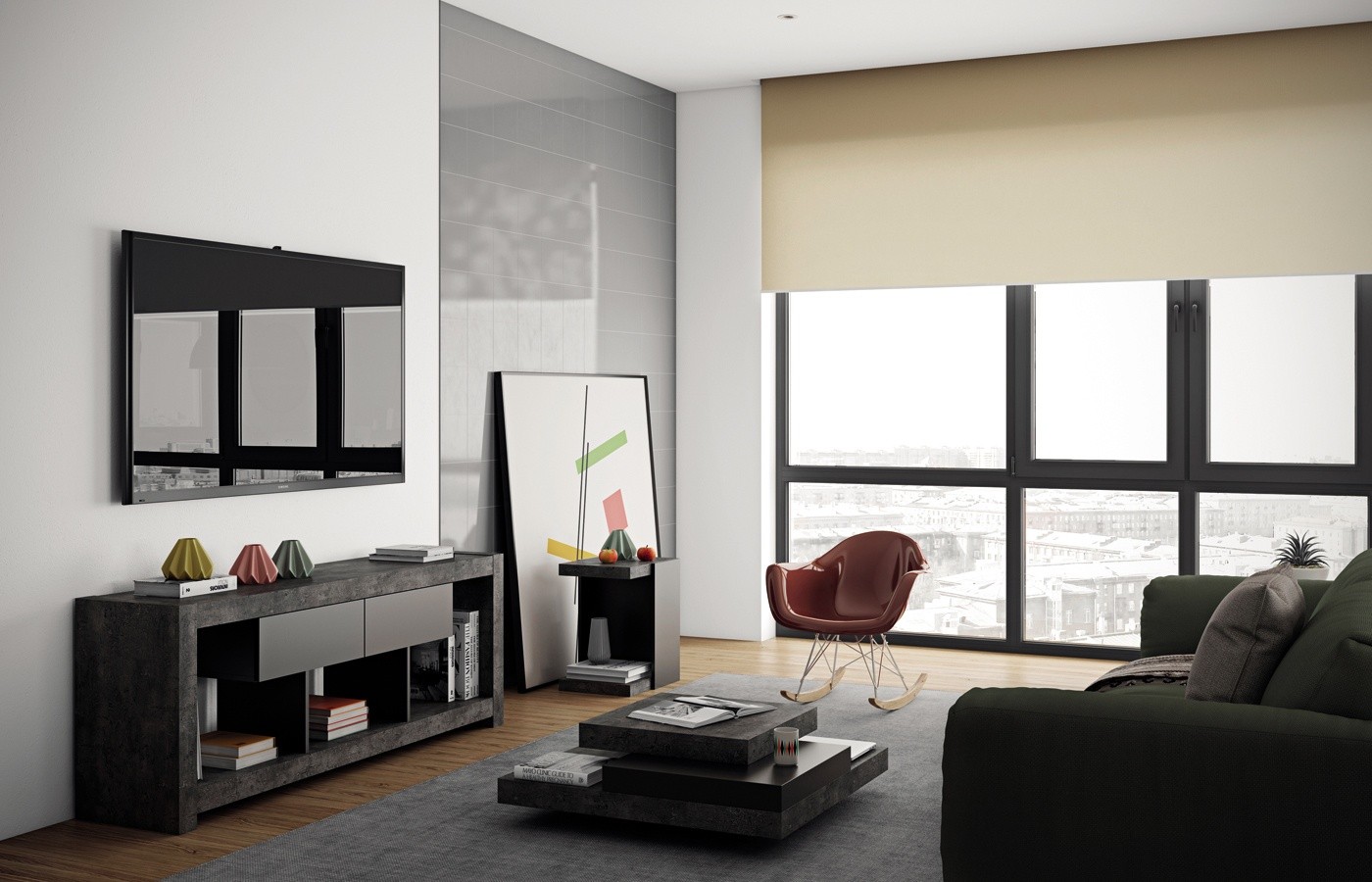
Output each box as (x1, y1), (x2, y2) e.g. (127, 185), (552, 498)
(627, 696), (777, 729)
(201, 731), (277, 770)
(368, 543), (455, 563)
(514, 752), (608, 787)
(565, 659), (651, 684)
(308, 695), (368, 741)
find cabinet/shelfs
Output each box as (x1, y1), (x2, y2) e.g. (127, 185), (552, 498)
(559, 553), (680, 698)
(74, 551), (517, 833)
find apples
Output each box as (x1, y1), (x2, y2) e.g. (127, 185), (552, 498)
(638, 545), (655, 560)
(599, 547), (618, 563)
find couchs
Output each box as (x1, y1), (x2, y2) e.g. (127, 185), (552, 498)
(939, 547), (1372, 882)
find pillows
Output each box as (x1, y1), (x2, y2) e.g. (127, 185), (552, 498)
(1185, 562), (1304, 702)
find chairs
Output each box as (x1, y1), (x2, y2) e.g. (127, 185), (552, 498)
(766, 531), (931, 709)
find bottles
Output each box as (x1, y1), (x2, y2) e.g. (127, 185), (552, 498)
(588, 617), (611, 665)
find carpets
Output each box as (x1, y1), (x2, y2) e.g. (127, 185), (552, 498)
(164, 671), (961, 882)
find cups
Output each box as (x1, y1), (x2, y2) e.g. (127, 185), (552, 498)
(774, 727), (799, 765)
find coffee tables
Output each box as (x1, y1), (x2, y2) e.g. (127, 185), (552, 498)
(497, 692), (888, 841)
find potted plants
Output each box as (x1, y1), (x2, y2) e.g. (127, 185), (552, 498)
(1272, 529), (1333, 579)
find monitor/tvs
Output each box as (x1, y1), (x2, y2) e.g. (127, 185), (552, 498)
(122, 230), (405, 507)
